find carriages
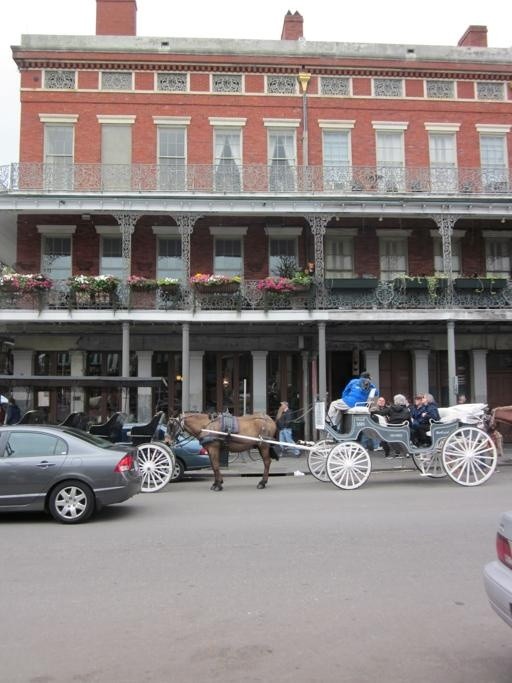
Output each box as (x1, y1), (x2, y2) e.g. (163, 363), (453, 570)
(164, 388), (498, 492)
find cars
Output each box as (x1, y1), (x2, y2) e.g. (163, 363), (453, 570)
(484, 511), (512, 628)
(121, 423), (225, 483)
(0, 424), (142, 524)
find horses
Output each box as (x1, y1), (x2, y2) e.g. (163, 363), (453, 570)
(163, 409), (278, 492)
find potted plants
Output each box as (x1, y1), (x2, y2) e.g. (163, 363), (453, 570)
(395, 272), (508, 299)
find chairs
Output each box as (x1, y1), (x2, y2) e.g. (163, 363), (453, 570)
(12, 408), (164, 447)
(343, 387), (376, 416)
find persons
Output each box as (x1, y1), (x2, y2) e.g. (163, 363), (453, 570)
(456, 392), (504, 475)
(277, 400), (302, 459)
(303, 261), (315, 276)
(1, 392), (22, 426)
(325, 371), (442, 459)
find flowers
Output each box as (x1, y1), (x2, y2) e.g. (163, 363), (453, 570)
(0, 262), (315, 293)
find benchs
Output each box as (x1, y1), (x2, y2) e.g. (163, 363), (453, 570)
(372, 407), (457, 450)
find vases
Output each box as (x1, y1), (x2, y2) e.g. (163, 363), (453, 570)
(324, 278), (378, 289)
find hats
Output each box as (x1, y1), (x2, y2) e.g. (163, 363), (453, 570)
(361, 372), (372, 380)
